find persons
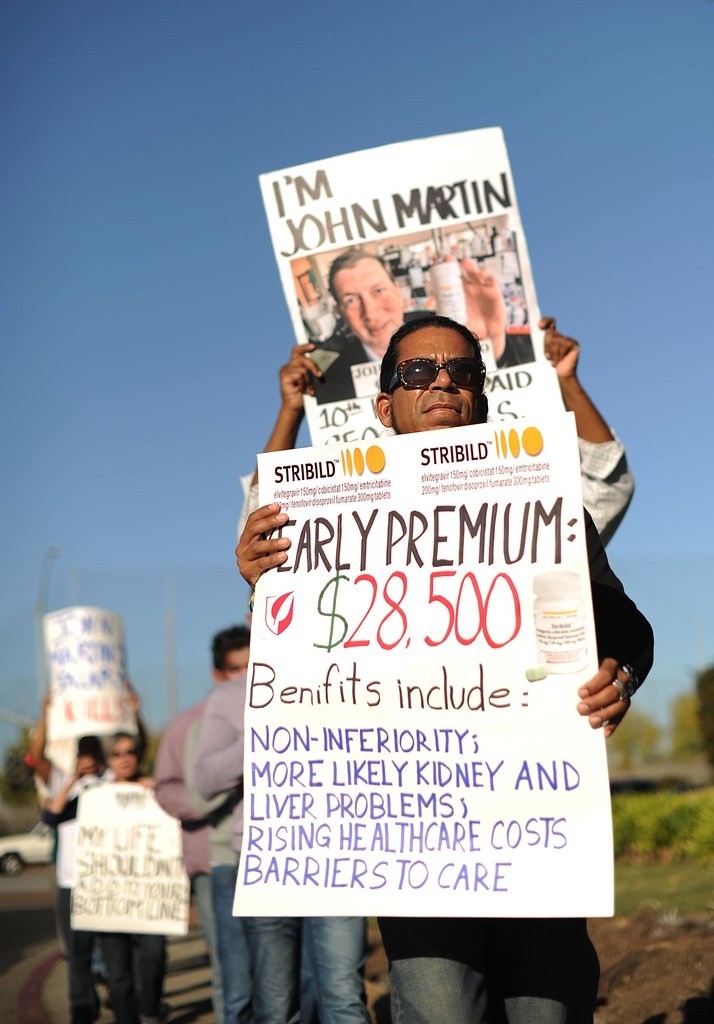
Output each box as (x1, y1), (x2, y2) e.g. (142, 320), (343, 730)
(304, 248), (518, 408)
(233, 312), (657, 1023)
(234, 315), (640, 559)
(25, 624), (377, 1024)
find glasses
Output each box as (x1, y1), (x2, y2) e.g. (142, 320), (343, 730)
(110, 748), (137, 757)
(387, 355), (486, 394)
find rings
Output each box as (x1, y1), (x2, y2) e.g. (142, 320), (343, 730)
(612, 679), (628, 699)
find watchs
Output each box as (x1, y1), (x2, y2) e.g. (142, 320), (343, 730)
(617, 660), (640, 697)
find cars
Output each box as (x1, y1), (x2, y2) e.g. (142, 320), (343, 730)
(0, 818), (57, 876)
